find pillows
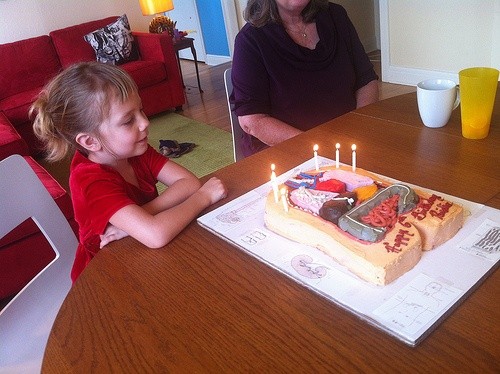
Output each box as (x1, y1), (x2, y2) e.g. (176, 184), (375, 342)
(83, 13), (141, 65)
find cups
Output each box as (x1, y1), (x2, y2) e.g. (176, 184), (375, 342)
(416, 78), (462, 128)
(458, 67), (500, 139)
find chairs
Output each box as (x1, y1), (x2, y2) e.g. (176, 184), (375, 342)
(224, 67), (262, 163)
(0, 154), (79, 374)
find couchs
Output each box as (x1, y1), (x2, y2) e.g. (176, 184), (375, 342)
(0, 13), (187, 299)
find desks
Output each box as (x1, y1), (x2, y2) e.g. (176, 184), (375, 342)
(40, 79), (500, 374)
(174, 37), (203, 93)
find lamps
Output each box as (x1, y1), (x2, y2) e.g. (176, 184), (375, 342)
(139, 0), (174, 19)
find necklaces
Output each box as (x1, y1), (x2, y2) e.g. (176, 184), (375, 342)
(284, 22), (307, 37)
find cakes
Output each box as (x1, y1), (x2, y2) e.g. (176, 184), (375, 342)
(264, 165), (463, 286)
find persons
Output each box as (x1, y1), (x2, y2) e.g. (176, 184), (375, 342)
(226, 0), (380, 159)
(28, 61), (228, 286)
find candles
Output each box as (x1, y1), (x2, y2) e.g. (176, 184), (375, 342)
(351, 144), (357, 172)
(280, 189), (288, 210)
(271, 163), (280, 200)
(312, 144), (319, 169)
(335, 143), (340, 168)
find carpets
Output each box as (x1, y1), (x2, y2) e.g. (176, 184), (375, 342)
(35, 112), (235, 197)
